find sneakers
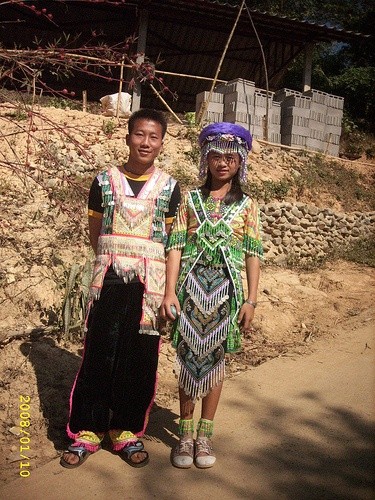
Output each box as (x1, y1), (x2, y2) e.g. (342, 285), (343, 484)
(195, 438), (216, 469)
(171, 436), (194, 468)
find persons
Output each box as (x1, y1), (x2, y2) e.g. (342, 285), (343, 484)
(160, 122), (264, 468)
(60, 109), (181, 469)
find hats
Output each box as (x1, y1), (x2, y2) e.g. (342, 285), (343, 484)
(198, 122), (252, 186)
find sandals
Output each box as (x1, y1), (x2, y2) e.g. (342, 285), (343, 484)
(60, 442), (104, 468)
(116, 439), (149, 468)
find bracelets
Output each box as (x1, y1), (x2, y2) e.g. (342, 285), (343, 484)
(246, 299), (257, 308)
